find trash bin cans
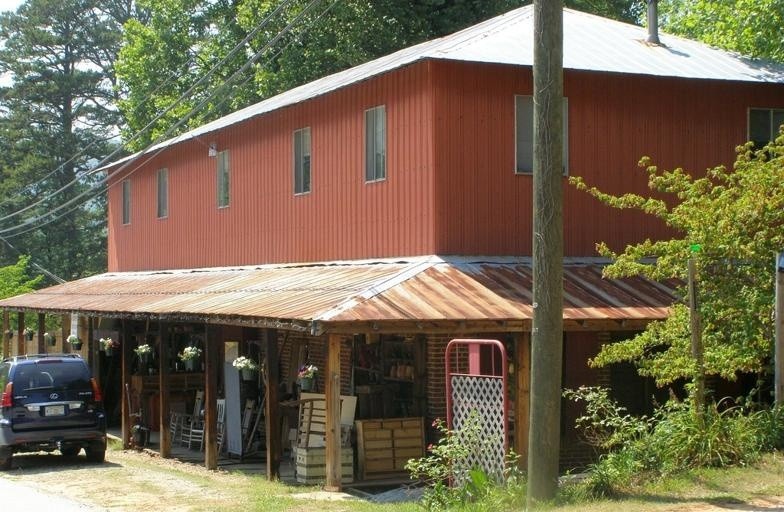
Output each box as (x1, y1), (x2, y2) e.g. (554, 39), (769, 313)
(130, 424), (151, 449)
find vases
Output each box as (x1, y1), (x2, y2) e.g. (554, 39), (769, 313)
(240, 367), (257, 382)
(103, 346), (112, 357)
(138, 353), (148, 365)
(183, 358), (196, 372)
(299, 376), (312, 390)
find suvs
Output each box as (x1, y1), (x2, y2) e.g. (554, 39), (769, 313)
(0, 352), (108, 471)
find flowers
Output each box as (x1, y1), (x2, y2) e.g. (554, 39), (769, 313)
(176, 346), (202, 361)
(97, 336), (119, 349)
(133, 344), (150, 355)
(297, 365), (318, 377)
(4, 327), (84, 351)
(231, 355), (262, 373)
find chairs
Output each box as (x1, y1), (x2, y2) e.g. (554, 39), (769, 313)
(168, 389), (256, 461)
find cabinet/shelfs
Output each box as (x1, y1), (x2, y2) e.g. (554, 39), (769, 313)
(353, 414), (426, 483)
(350, 333), (426, 420)
